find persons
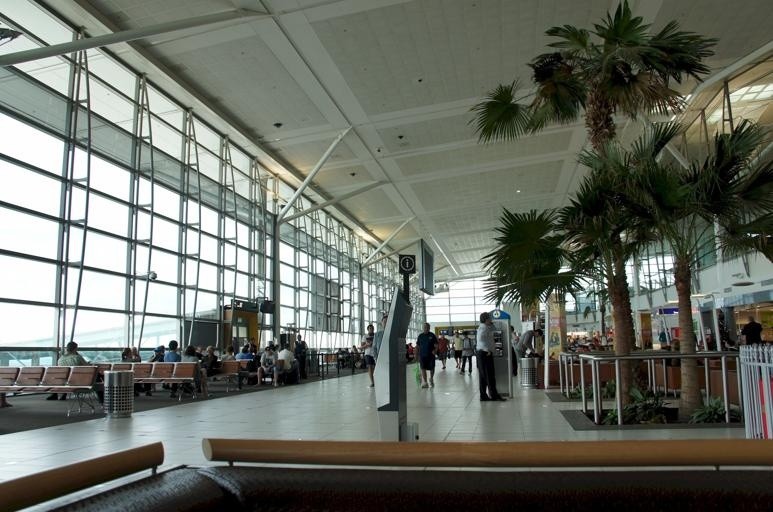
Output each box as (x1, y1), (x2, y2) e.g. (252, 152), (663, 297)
(515, 329), (543, 363)
(475, 312), (508, 402)
(407, 323), (451, 388)
(46, 341), (104, 405)
(659, 328), (671, 342)
(459, 331), (474, 376)
(741, 316), (763, 345)
(451, 332), (464, 369)
(510, 326), (519, 376)
(336, 316), (388, 387)
(121, 335), (308, 398)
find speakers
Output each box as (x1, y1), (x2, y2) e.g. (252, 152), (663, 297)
(260, 300), (275, 314)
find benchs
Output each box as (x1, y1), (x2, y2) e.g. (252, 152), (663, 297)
(0, 437), (773, 512)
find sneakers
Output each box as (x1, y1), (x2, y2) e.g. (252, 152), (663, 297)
(0, 401), (12, 407)
(442, 367), (445, 369)
(423, 379), (434, 388)
(456, 364), (471, 373)
(162, 384), (176, 398)
(146, 392), (152, 396)
(46, 395), (66, 401)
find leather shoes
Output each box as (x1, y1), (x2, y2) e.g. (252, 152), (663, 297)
(480, 397), (506, 401)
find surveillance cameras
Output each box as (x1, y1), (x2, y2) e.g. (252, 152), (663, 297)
(149, 272), (157, 281)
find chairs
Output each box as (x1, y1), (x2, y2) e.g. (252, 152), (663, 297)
(567, 357), (739, 405)
(0, 357), (299, 418)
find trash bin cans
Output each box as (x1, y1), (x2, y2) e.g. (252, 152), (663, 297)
(103, 369), (135, 418)
(519, 357), (540, 390)
